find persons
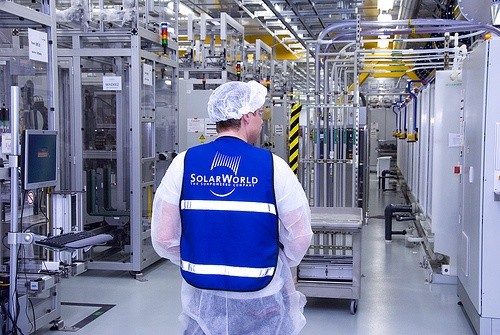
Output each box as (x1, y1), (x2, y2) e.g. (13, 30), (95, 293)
(150, 80), (313, 334)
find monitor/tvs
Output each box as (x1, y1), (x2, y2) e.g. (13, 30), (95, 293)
(21, 130), (60, 190)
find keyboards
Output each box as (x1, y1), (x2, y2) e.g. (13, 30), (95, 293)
(35, 232), (113, 248)
